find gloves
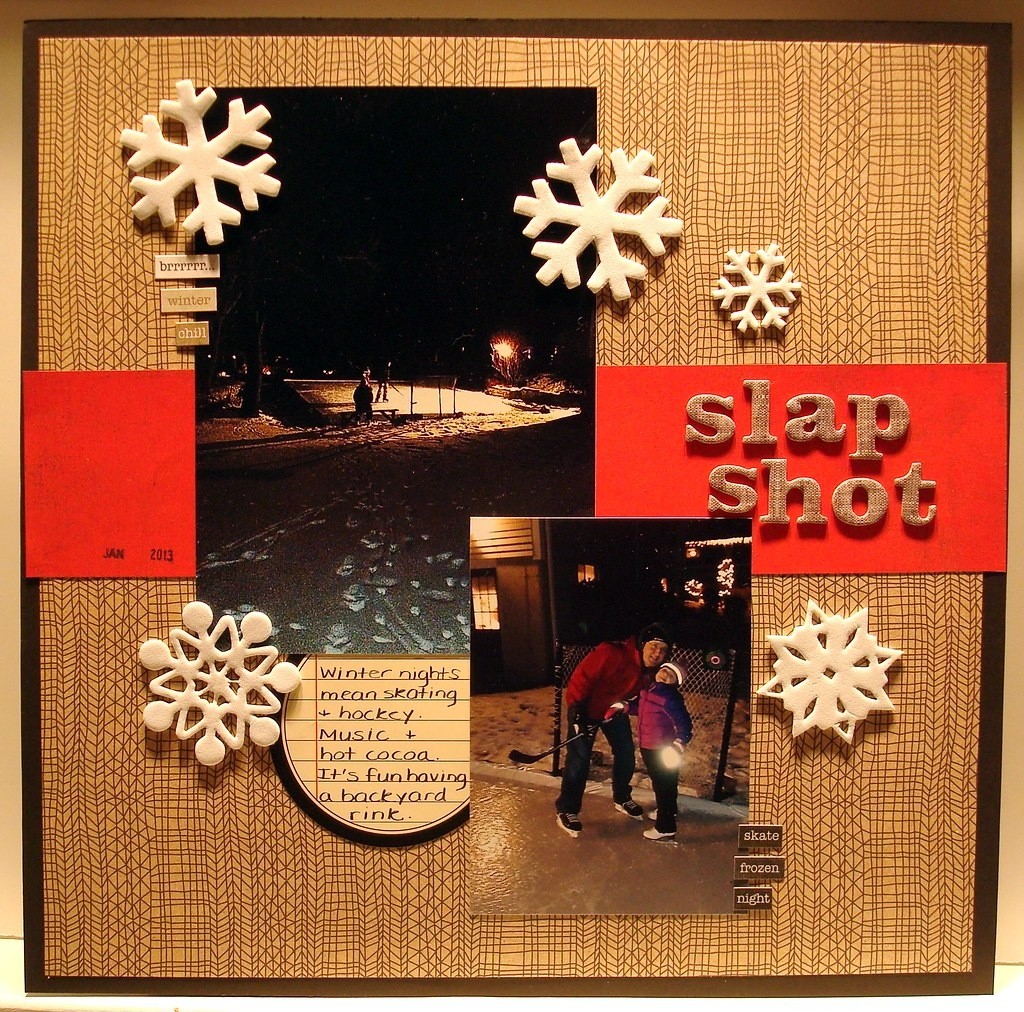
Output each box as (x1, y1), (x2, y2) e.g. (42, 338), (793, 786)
(569, 714), (596, 741)
(604, 701), (630, 721)
(662, 741), (684, 769)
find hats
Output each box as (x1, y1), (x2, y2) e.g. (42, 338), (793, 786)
(640, 623), (673, 652)
(660, 660), (690, 686)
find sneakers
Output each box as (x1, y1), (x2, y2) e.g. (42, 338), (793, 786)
(647, 808), (679, 822)
(555, 812), (583, 838)
(643, 825), (680, 845)
(613, 794), (645, 822)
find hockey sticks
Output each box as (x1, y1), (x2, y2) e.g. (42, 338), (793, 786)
(507, 712), (622, 764)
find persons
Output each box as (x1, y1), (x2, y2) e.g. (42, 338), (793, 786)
(609, 662), (696, 840)
(553, 622), (674, 839)
(353, 359), (393, 428)
